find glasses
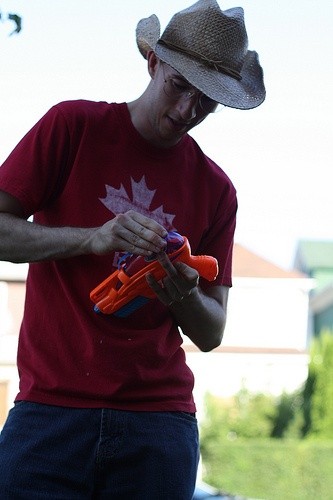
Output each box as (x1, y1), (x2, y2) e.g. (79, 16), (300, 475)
(160, 61), (223, 113)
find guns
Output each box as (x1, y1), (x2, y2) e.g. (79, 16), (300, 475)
(89, 230), (220, 321)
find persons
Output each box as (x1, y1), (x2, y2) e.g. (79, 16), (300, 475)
(0, 0), (268, 500)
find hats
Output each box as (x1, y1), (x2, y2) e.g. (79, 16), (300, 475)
(135, 0), (266, 110)
